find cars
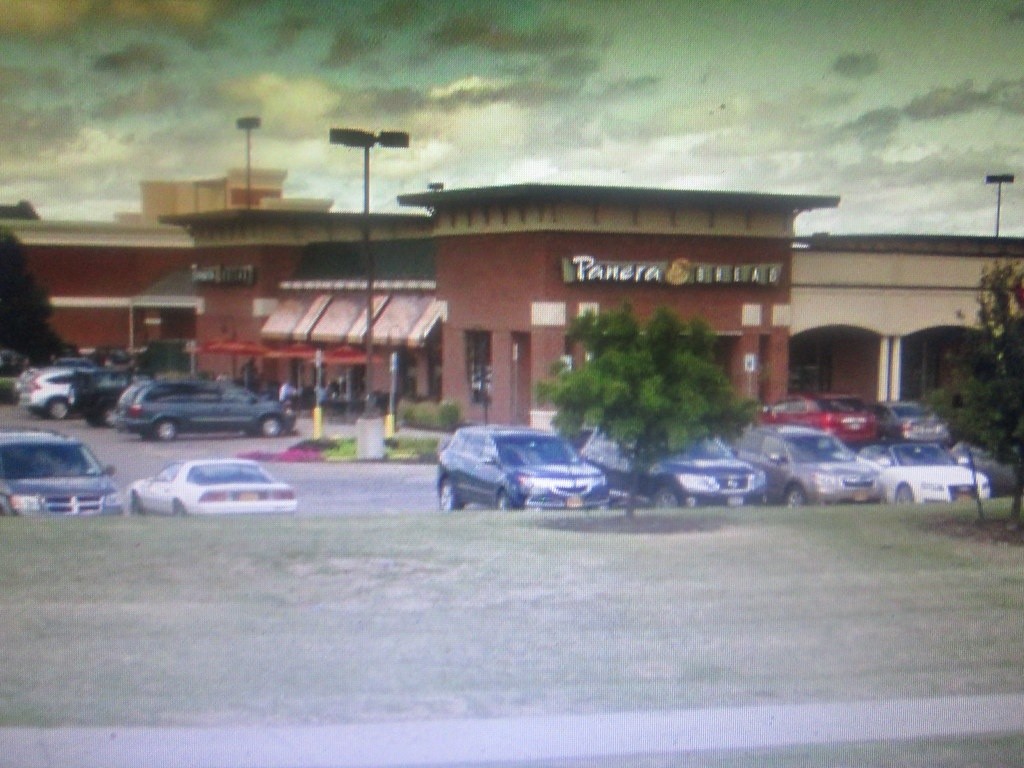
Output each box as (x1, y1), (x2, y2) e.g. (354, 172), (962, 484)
(17, 365), (103, 420)
(950, 440), (1023, 497)
(127, 458), (297, 515)
(88, 348), (127, 366)
(868, 401), (952, 447)
(755, 391), (877, 448)
(859, 439), (993, 506)
(0, 343), (94, 370)
(731, 423), (884, 507)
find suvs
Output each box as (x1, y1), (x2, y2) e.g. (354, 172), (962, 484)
(437, 424), (612, 511)
(582, 427), (768, 508)
(68, 368), (155, 427)
(110, 379), (297, 440)
(0, 425), (122, 516)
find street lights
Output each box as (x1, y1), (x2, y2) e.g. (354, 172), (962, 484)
(329, 126), (410, 419)
(236, 117), (261, 206)
(985, 176), (1015, 238)
